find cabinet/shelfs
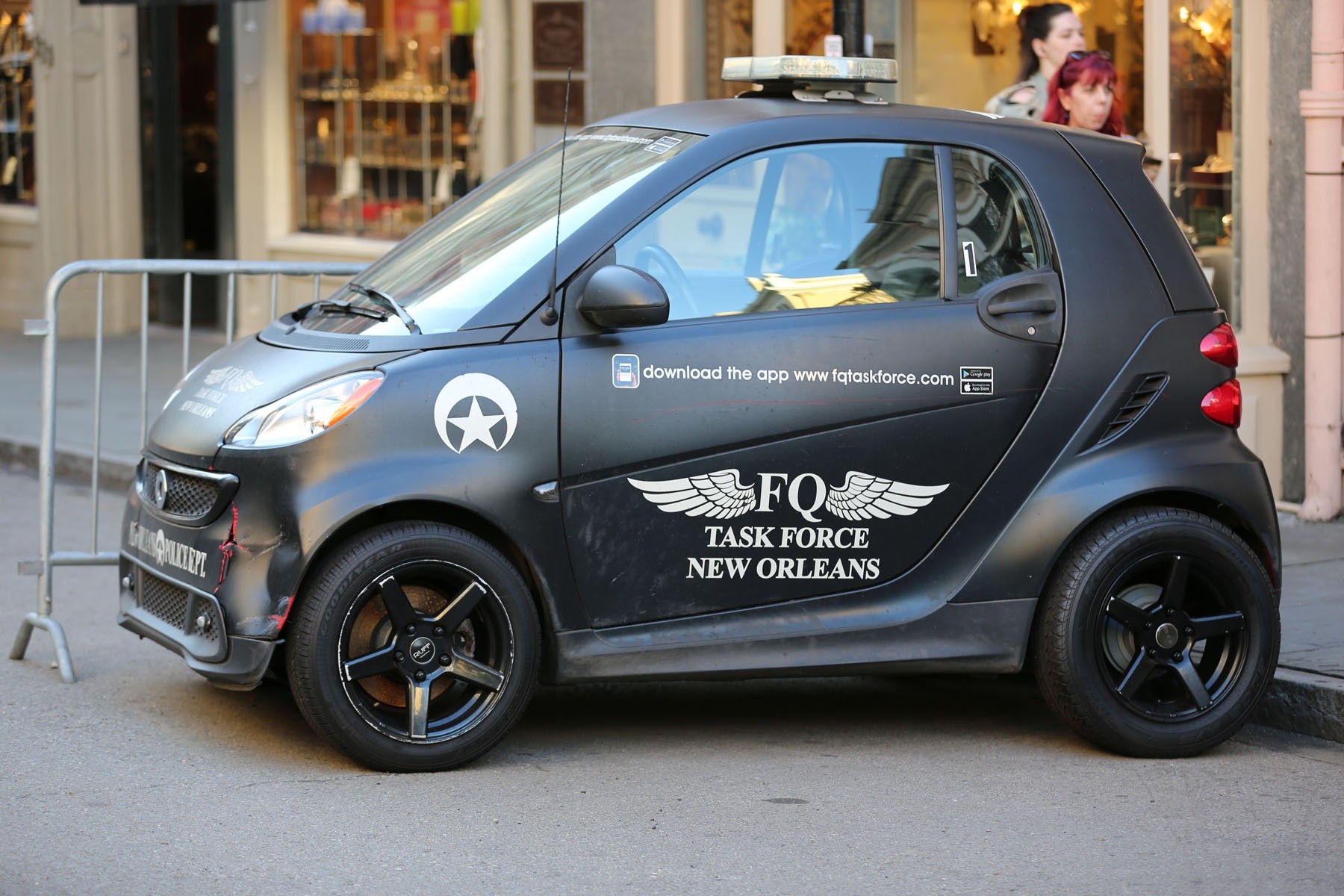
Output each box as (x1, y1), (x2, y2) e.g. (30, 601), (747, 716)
(294, 91), (473, 239)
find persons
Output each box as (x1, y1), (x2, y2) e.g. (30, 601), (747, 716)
(987, 3), (1087, 126)
(1044, 47), (1124, 141)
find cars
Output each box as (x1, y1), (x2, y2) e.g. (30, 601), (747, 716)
(120, 47), (1287, 763)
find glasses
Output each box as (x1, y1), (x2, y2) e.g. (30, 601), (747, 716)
(1060, 49), (1111, 84)
